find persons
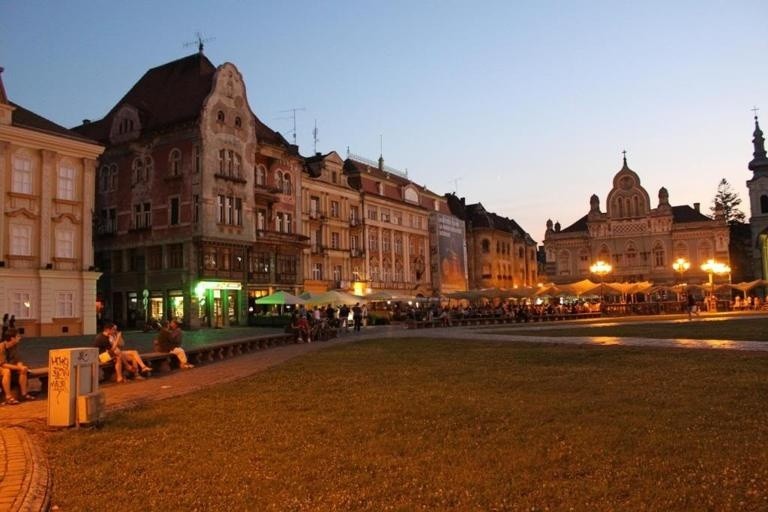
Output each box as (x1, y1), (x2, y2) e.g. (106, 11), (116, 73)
(9, 314), (17, 329)
(91, 323), (136, 386)
(730, 294), (768, 313)
(2, 313), (10, 340)
(155, 320), (196, 370)
(275, 302), (368, 343)
(105, 324), (154, 382)
(682, 288), (700, 320)
(168, 317), (183, 348)
(368, 297), (600, 329)
(1, 328), (35, 404)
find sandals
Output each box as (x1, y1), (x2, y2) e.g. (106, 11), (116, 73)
(6, 397), (19, 404)
(20, 394), (35, 401)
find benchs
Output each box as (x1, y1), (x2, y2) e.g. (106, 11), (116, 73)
(407, 313), (603, 329)
(1, 332), (297, 401)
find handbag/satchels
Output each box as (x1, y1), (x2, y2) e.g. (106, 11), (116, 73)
(99, 352), (111, 363)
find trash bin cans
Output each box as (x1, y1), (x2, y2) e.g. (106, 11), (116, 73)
(47, 347), (102, 428)
(716, 300), (729, 312)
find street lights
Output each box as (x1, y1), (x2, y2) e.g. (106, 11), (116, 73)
(589, 260), (612, 282)
(673, 258), (689, 283)
(713, 263), (732, 282)
(701, 260), (718, 286)
